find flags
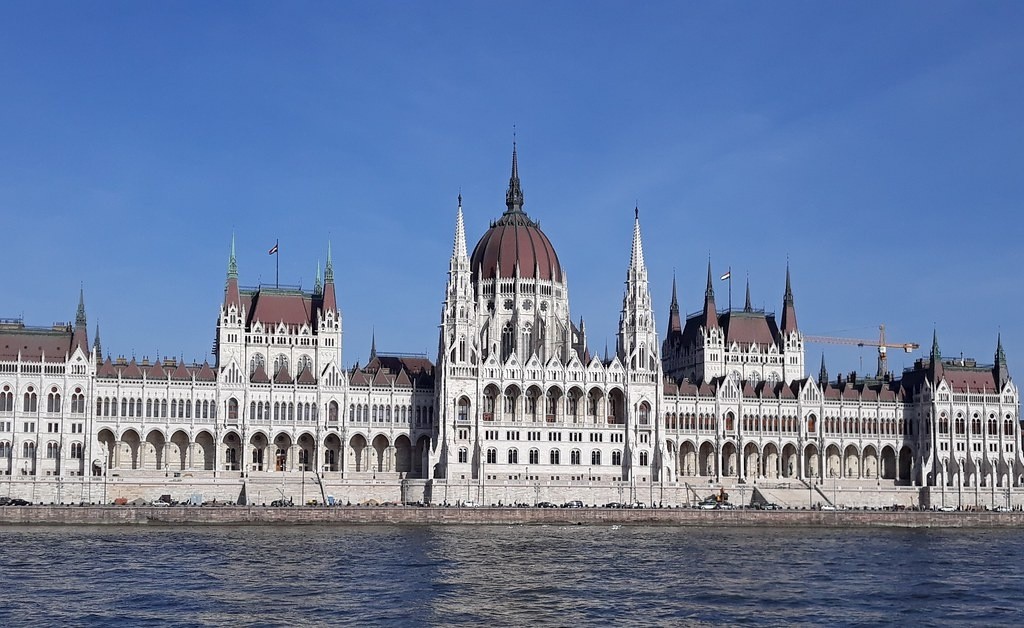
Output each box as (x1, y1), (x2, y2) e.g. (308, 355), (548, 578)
(720, 272), (730, 280)
(269, 246), (277, 255)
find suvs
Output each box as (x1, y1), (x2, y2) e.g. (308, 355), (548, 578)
(564, 500), (583, 508)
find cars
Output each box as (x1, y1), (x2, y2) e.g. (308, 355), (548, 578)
(761, 503), (781, 510)
(0, 496), (12, 506)
(152, 500), (170, 507)
(11, 499), (33, 506)
(700, 502), (717, 510)
(821, 505), (841, 511)
(535, 501), (557, 509)
(606, 502), (624, 509)
(994, 506), (1011, 512)
(939, 505), (955, 513)
(626, 502), (647, 508)
(462, 499), (480, 508)
(719, 502), (737, 510)
